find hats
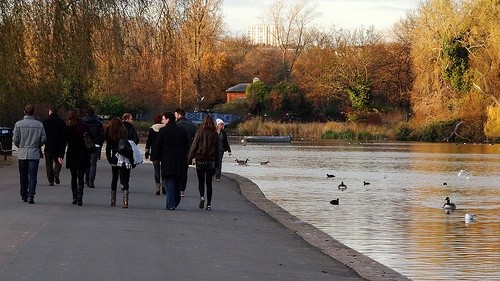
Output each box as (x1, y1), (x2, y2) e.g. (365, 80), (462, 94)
(86, 108), (96, 114)
(174, 108), (184, 114)
(216, 118), (225, 126)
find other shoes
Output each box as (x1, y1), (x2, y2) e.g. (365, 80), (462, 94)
(181, 192), (184, 197)
(205, 207), (211, 211)
(199, 197), (205, 209)
(217, 178), (221, 182)
(48, 182), (54, 186)
(86, 180), (95, 188)
(54, 176), (60, 184)
(23, 197), (34, 204)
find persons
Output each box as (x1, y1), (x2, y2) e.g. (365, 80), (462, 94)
(212, 119), (232, 181)
(175, 108), (195, 197)
(187, 115), (219, 211)
(13, 104), (47, 204)
(81, 108), (104, 188)
(58, 108), (91, 206)
(106, 117), (133, 208)
(120, 113), (139, 192)
(154, 112), (187, 210)
(42, 107), (66, 186)
(145, 116), (167, 195)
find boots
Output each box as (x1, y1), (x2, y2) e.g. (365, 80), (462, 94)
(72, 194), (83, 206)
(111, 190), (116, 207)
(162, 181), (167, 194)
(123, 190), (128, 208)
(155, 183), (161, 195)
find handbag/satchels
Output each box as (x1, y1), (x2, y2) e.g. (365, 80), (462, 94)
(118, 138), (132, 158)
(84, 131), (95, 150)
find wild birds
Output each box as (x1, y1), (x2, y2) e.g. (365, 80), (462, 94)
(443, 197), (456, 214)
(465, 214), (474, 220)
(235, 158), (249, 165)
(327, 174), (335, 178)
(447, 139), (496, 146)
(458, 169), (466, 176)
(363, 181), (370, 185)
(244, 144), (246, 146)
(338, 182), (347, 191)
(330, 198), (339, 205)
(259, 161), (270, 165)
(443, 182), (447, 185)
